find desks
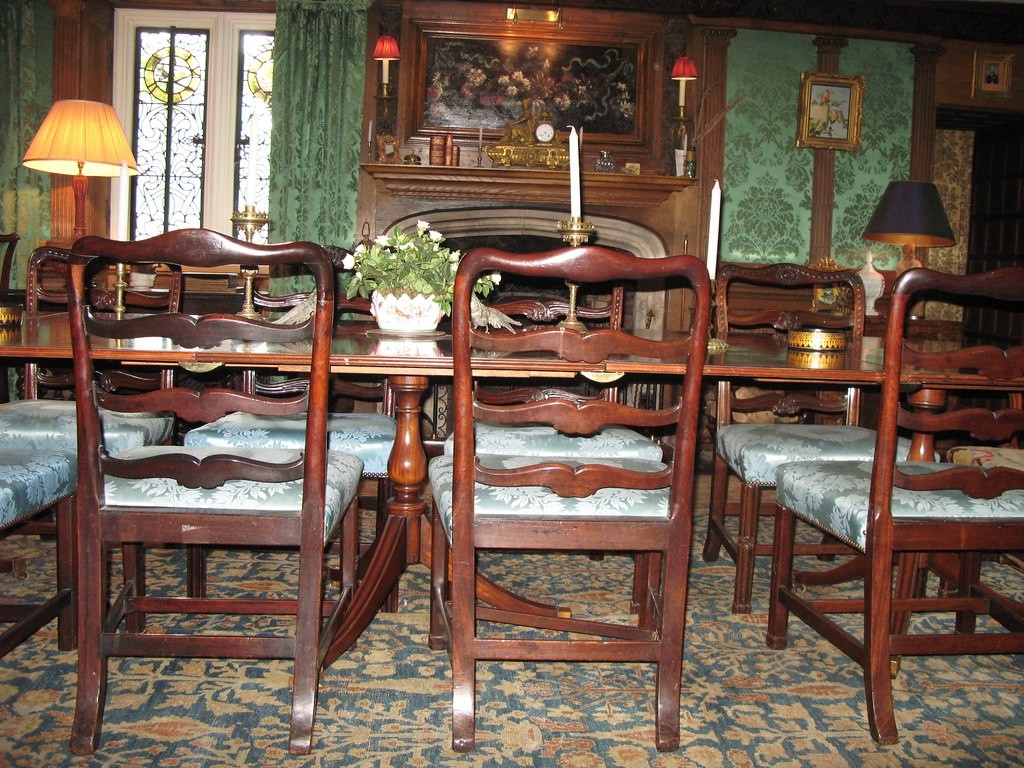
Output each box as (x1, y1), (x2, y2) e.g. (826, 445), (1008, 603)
(0, 309), (1024, 616)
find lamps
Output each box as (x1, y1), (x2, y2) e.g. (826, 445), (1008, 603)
(372, 35), (401, 134)
(863, 181), (957, 320)
(24, 98), (137, 241)
(670, 57), (698, 122)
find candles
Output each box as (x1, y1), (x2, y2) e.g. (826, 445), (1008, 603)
(705, 177), (721, 279)
(580, 126), (584, 148)
(565, 125), (584, 221)
(478, 125), (483, 147)
(368, 120), (373, 142)
(684, 132), (689, 156)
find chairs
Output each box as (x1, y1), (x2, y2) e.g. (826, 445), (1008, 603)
(0, 231), (1023, 752)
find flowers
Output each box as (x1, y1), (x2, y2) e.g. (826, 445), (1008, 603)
(340, 219), (502, 316)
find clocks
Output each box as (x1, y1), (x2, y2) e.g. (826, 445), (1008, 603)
(485, 100), (570, 169)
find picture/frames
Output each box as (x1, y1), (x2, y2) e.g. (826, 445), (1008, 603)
(973, 50), (1014, 98)
(796, 72), (866, 148)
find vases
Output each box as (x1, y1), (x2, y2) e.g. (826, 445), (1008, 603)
(373, 292), (445, 332)
